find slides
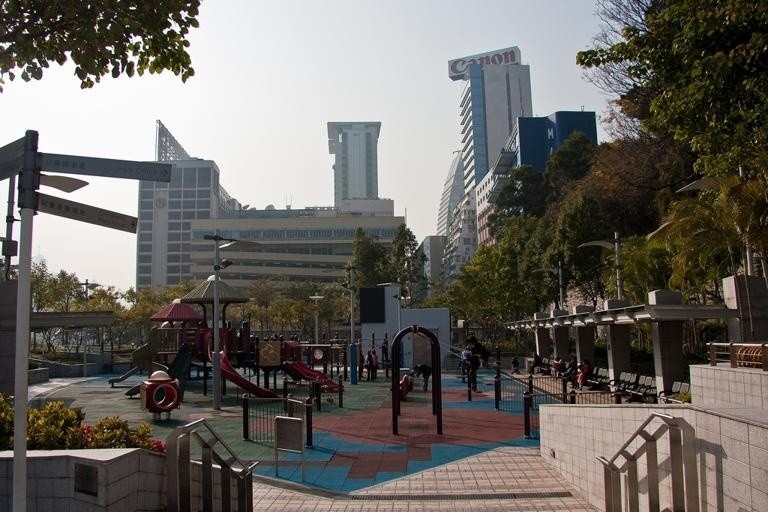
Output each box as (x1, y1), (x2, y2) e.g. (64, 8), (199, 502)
(283, 362), (344, 392)
(106, 342), (195, 395)
(207, 343), (280, 403)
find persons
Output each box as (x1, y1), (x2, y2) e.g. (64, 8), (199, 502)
(560, 355), (591, 390)
(529, 352), (543, 374)
(552, 355), (564, 381)
(411, 364), (433, 391)
(361, 350), (378, 380)
(460, 347), (470, 374)
(511, 356), (520, 375)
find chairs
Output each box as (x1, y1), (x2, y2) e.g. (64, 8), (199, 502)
(534, 357), (691, 404)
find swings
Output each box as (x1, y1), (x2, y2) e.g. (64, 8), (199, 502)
(415, 332), (485, 368)
